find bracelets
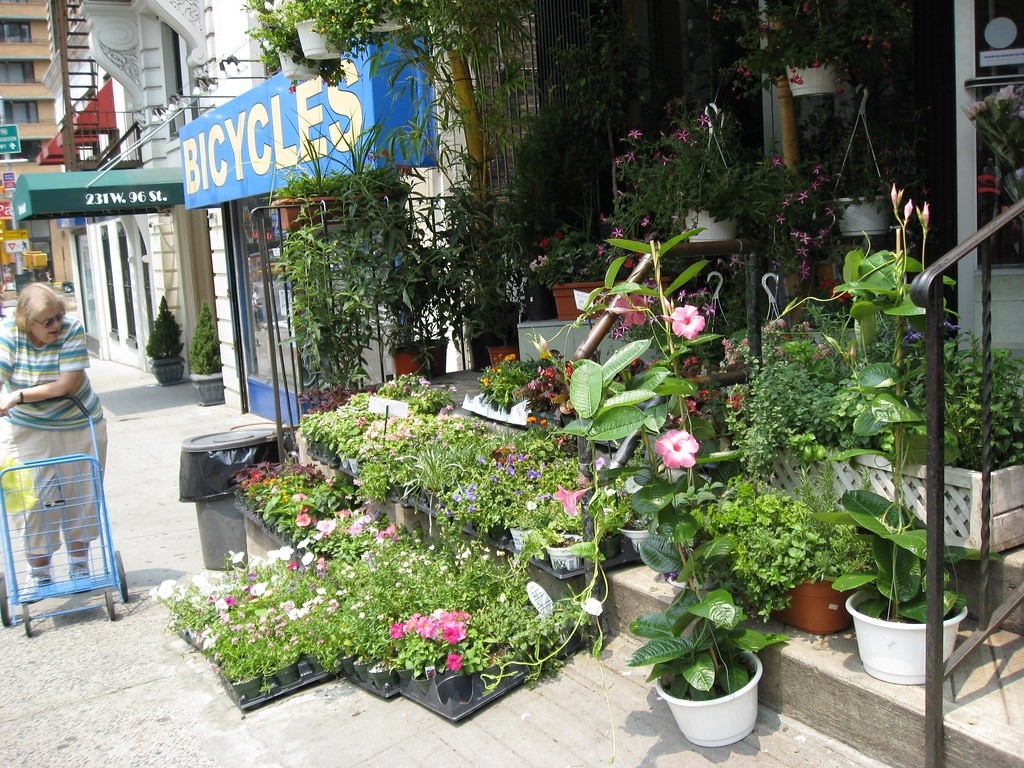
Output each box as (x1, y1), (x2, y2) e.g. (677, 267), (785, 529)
(20, 392), (24, 403)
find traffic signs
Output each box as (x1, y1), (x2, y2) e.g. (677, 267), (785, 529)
(0, 124), (22, 154)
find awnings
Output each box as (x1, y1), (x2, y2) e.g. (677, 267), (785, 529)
(37, 133), (80, 166)
(73, 79), (116, 134)
(56, 118), (98, 147)
(12, 167), (185, 222)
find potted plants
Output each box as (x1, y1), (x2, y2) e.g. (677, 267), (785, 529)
(363, 0), (405, 32)
(147, 294), (187, 387)
(474, 271), (522, 365)
(158, 0), (929, 678)
(699, 455), (873, 636)
(416, 294), (450, 377)
(388, 285), (424, 378)
(186, 299), (225, 406)
(240, 0), (320, 81)
(272, 170), (412, 232)
(290, 0), (342, 60)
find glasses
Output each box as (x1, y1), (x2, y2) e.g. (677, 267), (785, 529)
(33, 309), (64, 327)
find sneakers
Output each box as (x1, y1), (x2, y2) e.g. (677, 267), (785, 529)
(69, 565), (90, 594)
(18, 575), (51, 603)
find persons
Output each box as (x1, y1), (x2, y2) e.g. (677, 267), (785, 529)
(0, 283), (107, 602)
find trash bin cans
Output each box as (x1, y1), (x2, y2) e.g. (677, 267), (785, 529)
(182, 426), (282, 569)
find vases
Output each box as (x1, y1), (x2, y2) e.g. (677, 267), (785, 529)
(551, 275), (671, 321)
(829, 195), (891, 237)
(338, 657), (359, 676)
(394, 482), (408, 499)
(510, 525), (535, 551)
(425, 491), (438, 508)
(654, 647), (764, 747)
(348, 458), (357, 473)
(306, 654), (325, 673)
(545, 534), (581, 572)
(685, 207), (738, 242)
(844, 584), (968, 685)
(271, 659), (299, 688)
(367, 662), (397, 691)
(231, 674), (263, 701)
(525, 286), (556, 320)
(355, 656), (381, 681)
(395, 667), (414, 685)
(758, 463), (1024, 552)
(786, 62), (835, 98)
(619, 526), (657, 553)
(666, 542), (720, 591)
(487, 521), (507, 542)
(412, 675), (432, 695)
(338, 452), (348, 469)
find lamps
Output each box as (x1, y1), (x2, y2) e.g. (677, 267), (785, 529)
(219, 55), (269, 80)
(194, 72), (220, 94)
(166, 92), (237, 112)
(151, 105), (217, 117)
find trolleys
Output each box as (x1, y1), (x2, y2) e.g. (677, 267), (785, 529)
(0, 395), (131, 637)
